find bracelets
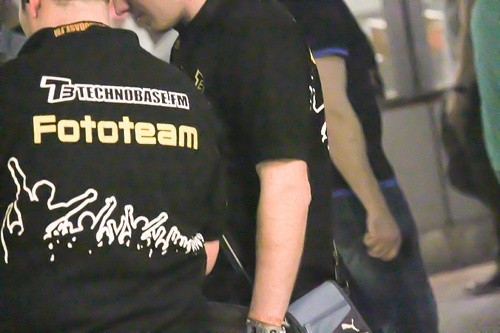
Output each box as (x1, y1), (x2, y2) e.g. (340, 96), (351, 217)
(246, 317), (286, 333)
(450, 82), (474, 97)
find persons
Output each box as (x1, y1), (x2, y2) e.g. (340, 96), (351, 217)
(0, 0), (500, 333)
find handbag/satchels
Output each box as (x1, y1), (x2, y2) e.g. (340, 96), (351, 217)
(286, 279), (373, 332)
